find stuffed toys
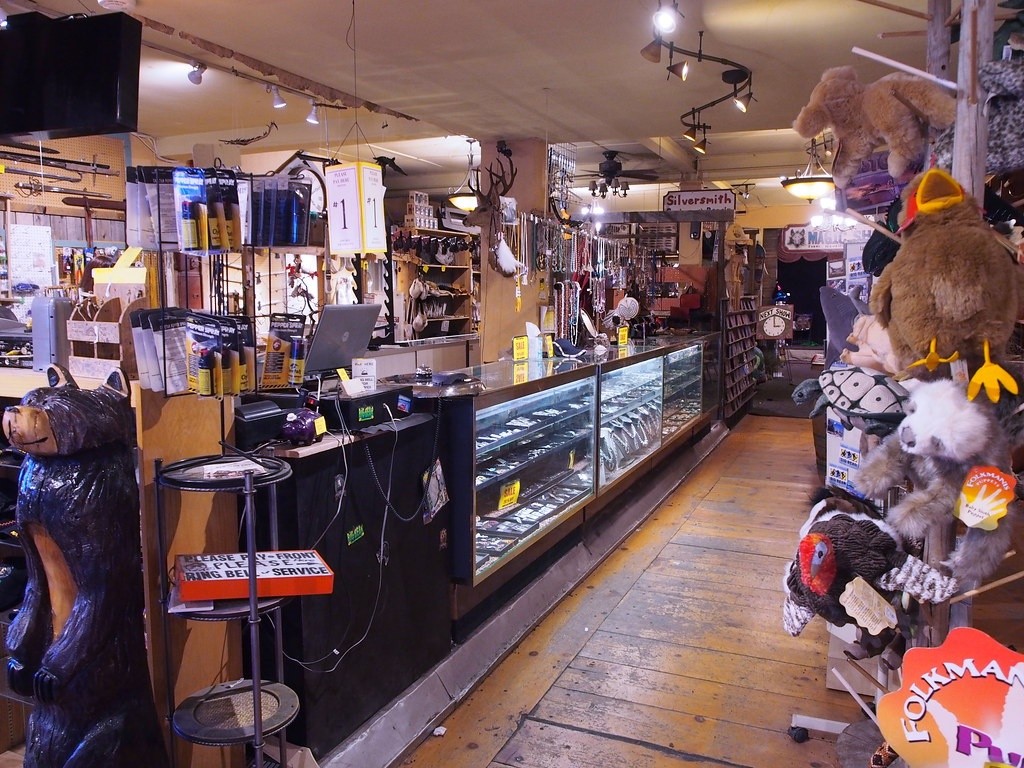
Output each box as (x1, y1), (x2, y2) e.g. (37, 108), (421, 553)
(781, 13), (1024, 670)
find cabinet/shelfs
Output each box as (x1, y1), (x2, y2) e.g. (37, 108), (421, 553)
(475, 343), (704, 572)
(610, 264), (715, 323)
(387, 227), (481, 346)
(0, 284), (299, 767)
(722, 295), (758, 430)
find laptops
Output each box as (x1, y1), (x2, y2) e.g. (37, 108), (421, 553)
(303, 304), (382, 374)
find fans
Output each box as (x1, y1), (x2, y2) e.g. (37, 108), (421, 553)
(569, 150), (660, 183)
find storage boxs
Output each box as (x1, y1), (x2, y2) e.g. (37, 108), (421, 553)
(405, 191), (438, 229)
(174, 549), (333, 600)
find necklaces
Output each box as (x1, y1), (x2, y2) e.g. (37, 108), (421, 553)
(649, 414), (660, 438)
(613, 432), (630, 454)
(632, 424), (649, 447)
(641, 419), (653, 443)
(599, 438), (618, 470)
(510, 211), (650, 348)
(616, 419), (639, 450)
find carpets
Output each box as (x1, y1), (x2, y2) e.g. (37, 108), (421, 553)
(747, 363), (824, 417)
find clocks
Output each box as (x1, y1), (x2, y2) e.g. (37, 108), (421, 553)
(755, 305), (793, 340)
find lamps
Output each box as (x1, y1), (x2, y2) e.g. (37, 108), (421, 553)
(587, 178), (630, 200)
(305, 99), (320, 125)
(187, 60), (207, 86)
(780, 135), (834, 203)
(810, 197), (858, 234)
(448, 138), (479, 213)
(640, 0), (758, 152)
(262, 83), (286, 107)
